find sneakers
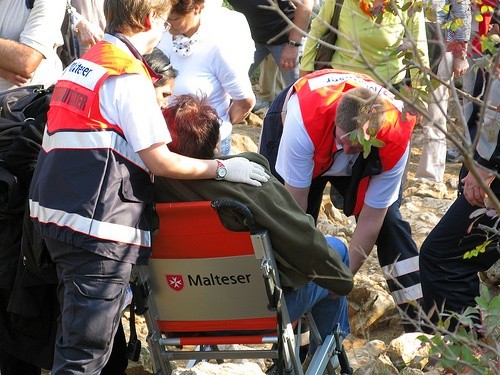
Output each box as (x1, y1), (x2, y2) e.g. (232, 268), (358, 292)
(302, 354), (340, 374)
(446, 149), (464, 163)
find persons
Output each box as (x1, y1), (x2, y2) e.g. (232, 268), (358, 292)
(259, 68), (430, 333)
(0, 1), (270, 375)
(281, 0), (500, 340)
(152, 89), (355, 375)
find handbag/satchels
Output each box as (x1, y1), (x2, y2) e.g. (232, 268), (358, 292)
(0, 85), (49, 129)
(58, 7), (82, 68)
(314, 0), (345, 70)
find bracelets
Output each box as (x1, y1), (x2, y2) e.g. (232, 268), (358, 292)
(458, 54), (468, 60)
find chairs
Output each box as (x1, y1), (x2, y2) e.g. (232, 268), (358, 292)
(135, 200), (354, 375)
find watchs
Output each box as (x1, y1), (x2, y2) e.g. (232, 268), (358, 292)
(215, 159), (227, 181)
(286, 38), (303, 47)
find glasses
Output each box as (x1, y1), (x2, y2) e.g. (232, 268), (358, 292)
(156, 10), (171, 32)
